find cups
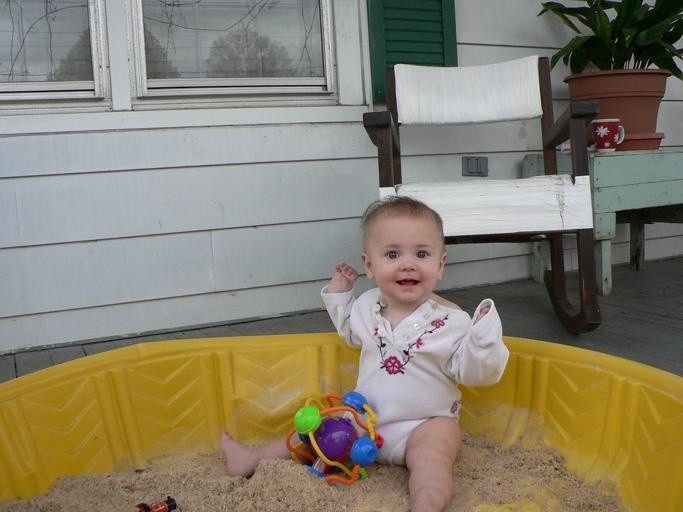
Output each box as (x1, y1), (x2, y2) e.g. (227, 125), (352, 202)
(588, 118), (625, 154)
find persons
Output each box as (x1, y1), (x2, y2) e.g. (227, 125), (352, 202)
(219, 195), (509, 511)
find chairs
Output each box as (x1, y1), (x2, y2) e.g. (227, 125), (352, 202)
(361, 51), (605, 341)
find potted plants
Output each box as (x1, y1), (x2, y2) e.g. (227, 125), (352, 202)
(532, 0), (682, 150)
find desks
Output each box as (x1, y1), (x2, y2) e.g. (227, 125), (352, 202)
(521, 144), (682, 299)
